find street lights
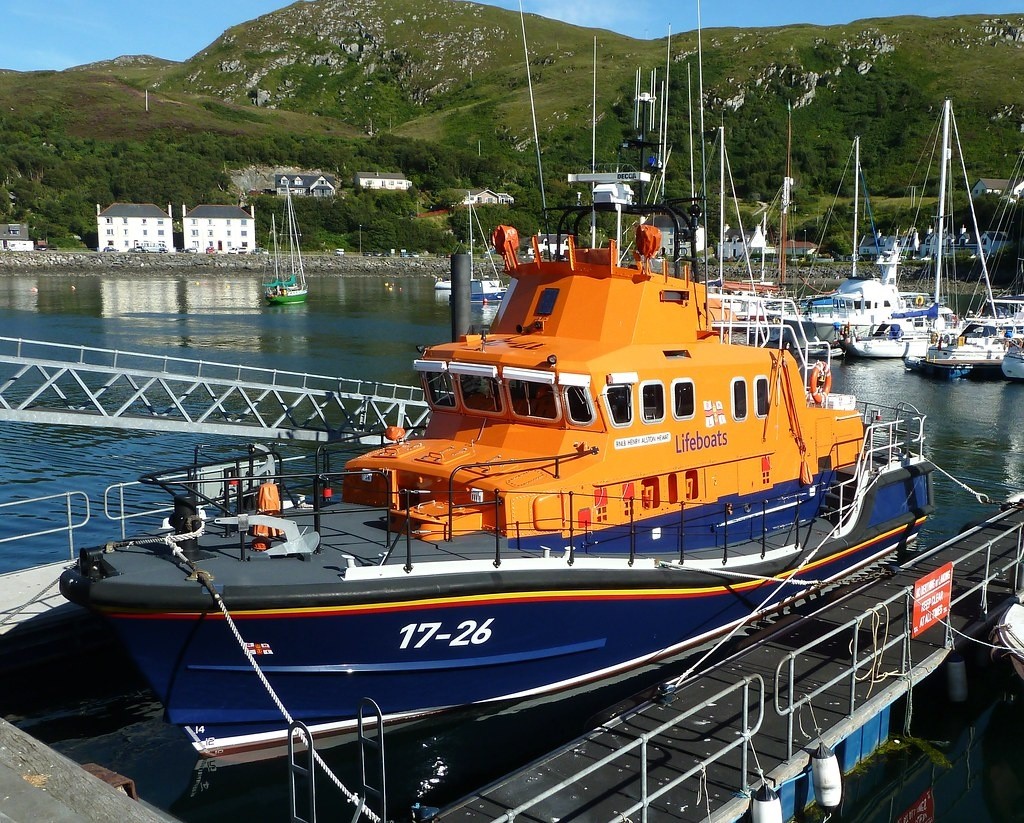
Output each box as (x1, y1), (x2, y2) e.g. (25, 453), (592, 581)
(360, 224), (362, 256)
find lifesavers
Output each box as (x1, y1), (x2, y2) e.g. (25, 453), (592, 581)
(810, 361), (832, 403)
(916, 295), (924, 305)
(958, 336), (964, 345)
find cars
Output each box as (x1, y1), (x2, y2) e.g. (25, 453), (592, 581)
(362, 250), (420, 258)
(34, 245), (47, 251)
(102, 244), (270, 254)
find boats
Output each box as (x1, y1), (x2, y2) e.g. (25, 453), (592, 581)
(448, 203), (508, 304)
(57, 1), (940, 795)
(432, 190), (482, 290)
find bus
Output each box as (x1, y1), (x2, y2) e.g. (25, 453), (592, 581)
(334, 248), (344, 256)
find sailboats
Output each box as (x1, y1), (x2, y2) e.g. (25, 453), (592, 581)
(259, 181), (309, 307)
(695, 97), (1023, 381)
